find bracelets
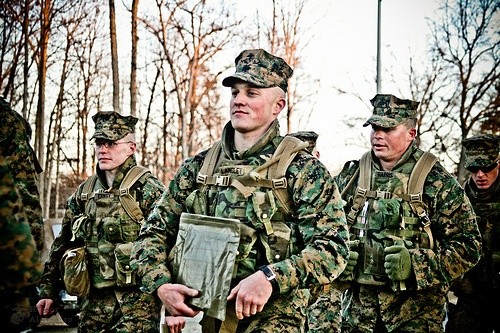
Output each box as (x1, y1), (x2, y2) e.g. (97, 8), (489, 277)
(259, 265), (280, 302)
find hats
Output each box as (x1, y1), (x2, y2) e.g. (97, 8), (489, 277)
(461, 134), (500, 167)
(89, 110), (139, 141)
(286, 131), (318, 153)
(222, 48), (294, 93)
(363, 93), (420, 128)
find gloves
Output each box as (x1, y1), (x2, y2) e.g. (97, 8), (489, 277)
(341, 239), (360, 274)
(383, 245), (412, 279)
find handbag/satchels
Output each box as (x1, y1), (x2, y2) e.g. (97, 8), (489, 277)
(60, 248), (90, 297)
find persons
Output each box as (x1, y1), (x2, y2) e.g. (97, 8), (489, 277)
(444, 133), (500, 333)
(131, 48), (350, 333)
(0, 93), (57, 333)
(284, 129), (320, 159)
(36, 110), (187, 333)
(329, 93), (484, 333)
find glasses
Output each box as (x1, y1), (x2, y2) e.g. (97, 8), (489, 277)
(91, 140), (133, 149)
(467, 158), (500, 174)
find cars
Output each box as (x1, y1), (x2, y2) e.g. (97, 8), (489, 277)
(43, 218), (80, 329)
